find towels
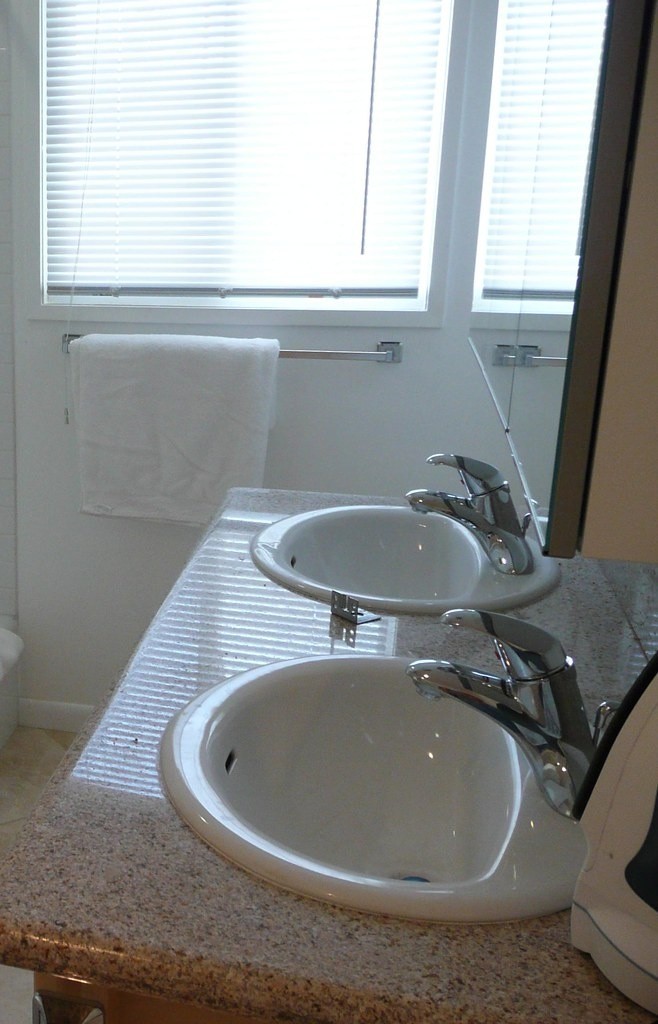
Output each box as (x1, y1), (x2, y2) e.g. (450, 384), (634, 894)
(67, 332), (280, 530)
(0, 627), (26, 679)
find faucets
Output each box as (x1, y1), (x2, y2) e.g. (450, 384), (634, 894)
(403, 608), (621, 818)
(404, 452), (536, 576)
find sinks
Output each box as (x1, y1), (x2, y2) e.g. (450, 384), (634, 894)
(247, 504), (560, 607)
(158, 654), (594, 925)
